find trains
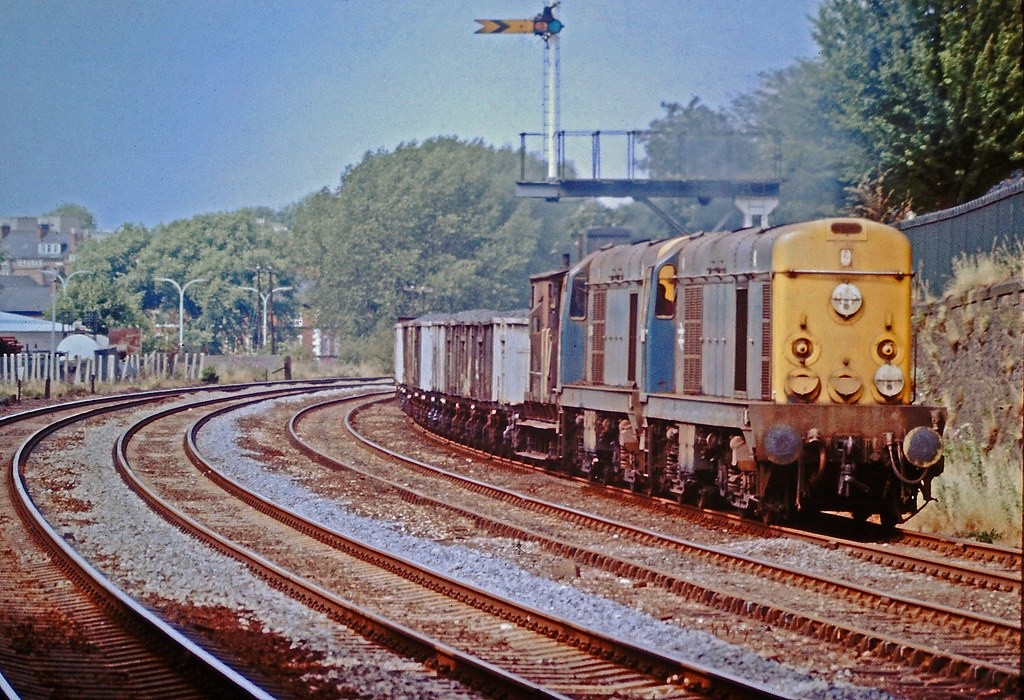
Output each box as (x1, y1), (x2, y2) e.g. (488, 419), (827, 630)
(397, 216), (948, 533)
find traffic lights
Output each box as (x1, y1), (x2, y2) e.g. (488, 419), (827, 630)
(535, 19), (563, 34)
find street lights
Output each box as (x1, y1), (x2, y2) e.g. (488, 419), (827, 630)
(238, 284), (297, 350)
(148, 278), (209, 352)
(40, 269), (94, 305)
